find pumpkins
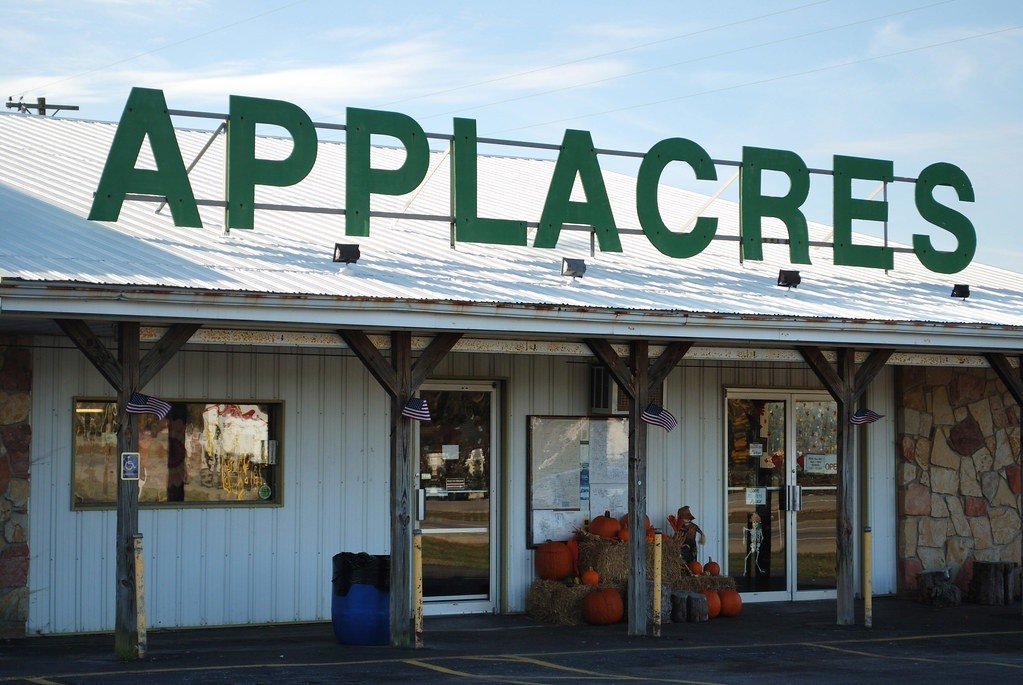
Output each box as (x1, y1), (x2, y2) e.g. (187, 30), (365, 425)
(535, 510), (742, 626)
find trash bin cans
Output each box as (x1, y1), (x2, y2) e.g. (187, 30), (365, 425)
(332, 550), (391, 646)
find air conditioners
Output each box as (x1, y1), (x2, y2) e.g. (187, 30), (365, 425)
(591, 364), (668, 416)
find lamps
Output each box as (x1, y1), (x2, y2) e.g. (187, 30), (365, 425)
(561, 257), (587, 280)
(952, 284), (970, 301)
(777, 269), (802, 290)
(332, 242), (361, 266)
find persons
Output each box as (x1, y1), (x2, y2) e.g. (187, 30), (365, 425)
(673, 506), (705, 577)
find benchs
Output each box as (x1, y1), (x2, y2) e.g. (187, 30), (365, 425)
(425, 487), (448, 501)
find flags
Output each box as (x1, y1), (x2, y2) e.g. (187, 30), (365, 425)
(850, 406), (886, 425)
(640, 401), (676, 432)
(402, 398), (432, 421)
(125, 392), (171, 421)
